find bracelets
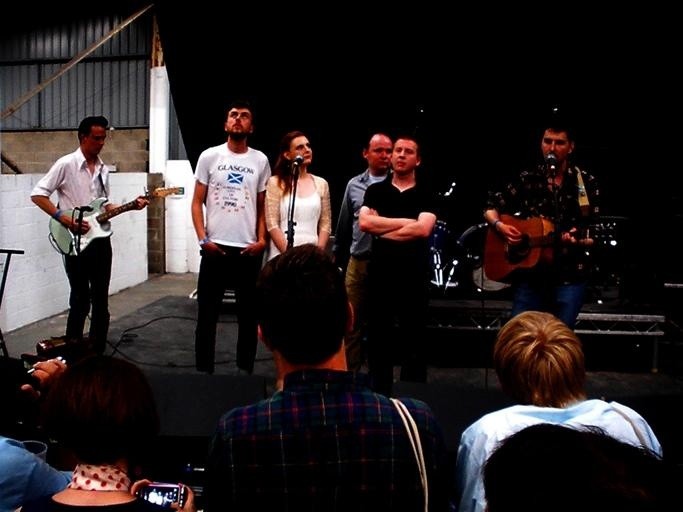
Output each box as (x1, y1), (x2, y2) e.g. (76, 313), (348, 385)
(199, 236), (208, 247)
(51, 209), (61, 219)
(490, 218), (501, 229)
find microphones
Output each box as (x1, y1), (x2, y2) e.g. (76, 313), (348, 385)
(546, 151), (559, 178)
(75, 204), (97, 217)
(293, 154), (304, 175)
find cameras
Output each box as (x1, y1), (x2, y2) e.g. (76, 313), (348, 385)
(138, 481), (184, 512)
(26, 354), (69, 389)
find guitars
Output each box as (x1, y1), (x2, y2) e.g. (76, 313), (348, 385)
(49, 187), (185, 257)
(483, 213), (618, 285)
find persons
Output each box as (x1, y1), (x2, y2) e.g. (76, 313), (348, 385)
(31, 115), (152, 355)
(482, 121), (605, 333)
(192, 103), (272, 375)
(0, 241), (683, 511)
(263, 129), (332, 393)
(358, 135), (439, 398)
(333, 130), (394, 351)
